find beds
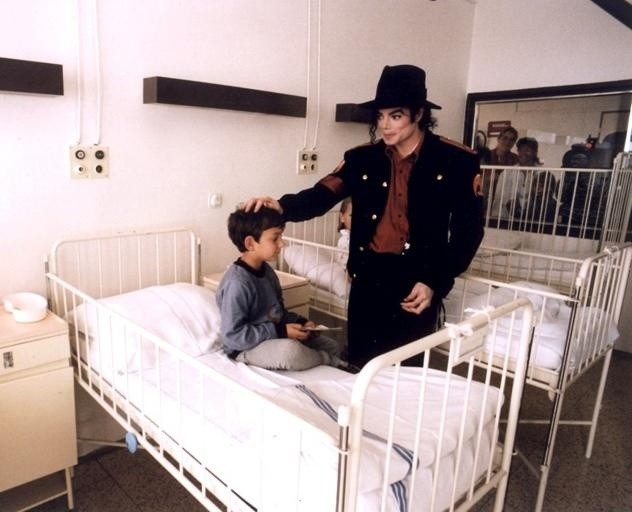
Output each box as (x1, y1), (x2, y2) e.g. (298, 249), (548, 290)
(45, 227), (536, 512)
(281, 207), (632, 512)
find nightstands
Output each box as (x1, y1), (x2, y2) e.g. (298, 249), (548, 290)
(2, 301), (79, 512)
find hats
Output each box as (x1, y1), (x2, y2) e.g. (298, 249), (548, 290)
(358, 64), (443, 111)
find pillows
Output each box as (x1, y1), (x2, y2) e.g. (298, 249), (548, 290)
(63, 281), (224, 374)
(283, 244), (350, 298)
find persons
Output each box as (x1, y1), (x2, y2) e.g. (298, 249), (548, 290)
(234, 64), (487, 366)
(474, 128), (631, 243)
(335, 196), (352, 269)
(214, 209), (360, 375)
